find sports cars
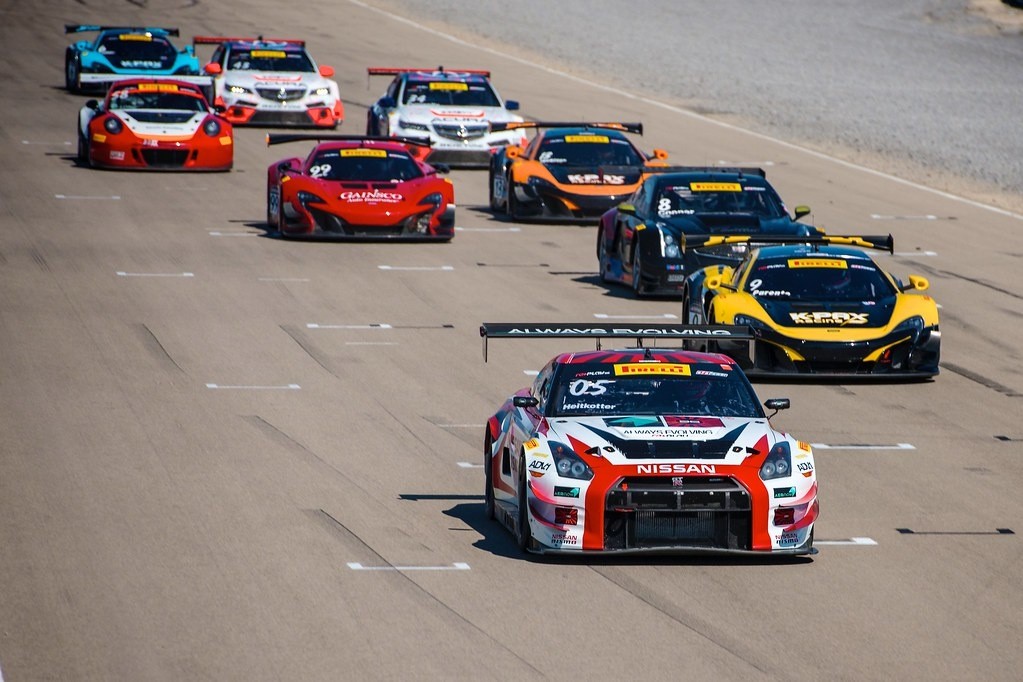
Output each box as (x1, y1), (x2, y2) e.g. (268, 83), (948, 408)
(63, 24), (203, 92)
(365, 66), (526, 165)
(477, 321), (822, 559)
(265, 132), (457, 241)
(74, 74), (234, 172)
(595, 166), (829, 297)
(678, 231), (944, 380)
(190, 35), (344, 130)
(487, 120), (671, 220)
